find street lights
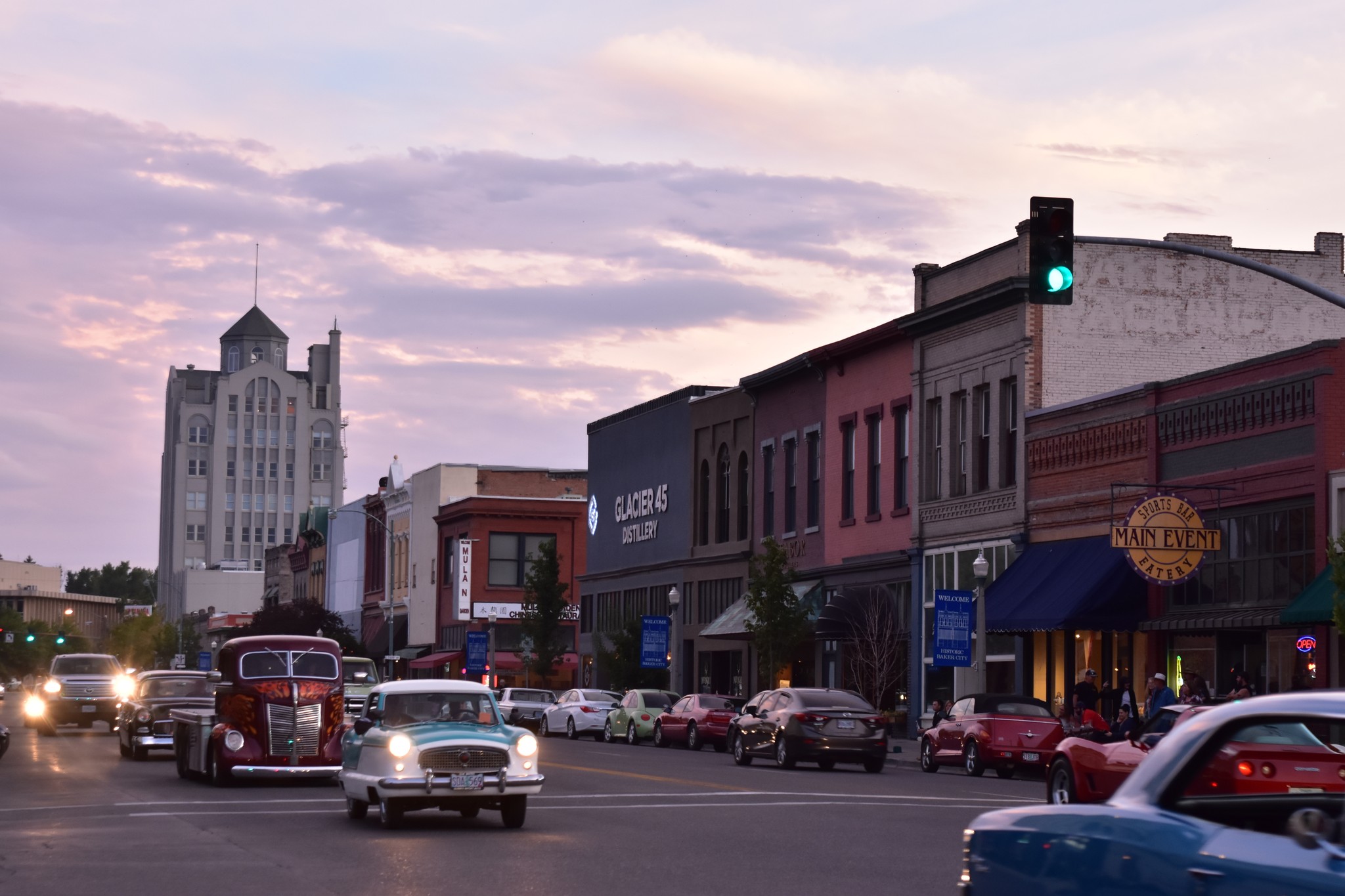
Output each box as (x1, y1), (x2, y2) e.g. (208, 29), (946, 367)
(211, 640), (220, 669)
(488, 611), (507, 692)
(327, 508), (395, 682)
(972, 548), (992, 692)
(669, 587), (682, 692)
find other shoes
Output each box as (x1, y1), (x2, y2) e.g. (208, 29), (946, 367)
(916, 757), (921, 760)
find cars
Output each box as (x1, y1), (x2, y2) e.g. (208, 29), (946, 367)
(726, 691), (773, 753)
(604, 689), (690, 746)
(959, 692), (1345, 891)
(484, 688), (559, 724)
(7, 680), (25, 692)
(734, 687), (886, 772)
(439, 690), (503, 719)
(1046, 705), (1345, 827)
(921, 694), (1065, 779)
(117, 670), (214, 762)
(337, 678), (544, 831)
(540, 689), (627, 741)
(655, 694), (747, 752)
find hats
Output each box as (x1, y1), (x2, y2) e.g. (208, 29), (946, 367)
(1154, 673), (1166, 680)
(1085, 670), (1098, 677)
(1181, 667), (1196, 674)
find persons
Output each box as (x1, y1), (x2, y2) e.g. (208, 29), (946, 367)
(915, 700), (955, 761)
(1070, 668), (1254, 741)
(140, 681), (164, 699)
(185, 680), (215, 699)
(300, 662), (321, 678)
(437, 700), (475, 724)
(344, 665), (376, 684)
(383, 699), (416, 728)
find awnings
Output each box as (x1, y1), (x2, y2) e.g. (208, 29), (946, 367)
(697, 578), (869, 642)
(1280, 562), (1337, 623)
(932, 534), (1144, 639)
(393, 646), (581, 673)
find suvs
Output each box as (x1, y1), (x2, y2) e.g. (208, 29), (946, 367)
(36, 653), (129, 736)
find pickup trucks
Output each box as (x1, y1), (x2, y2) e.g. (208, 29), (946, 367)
(170, 634), (357, 787)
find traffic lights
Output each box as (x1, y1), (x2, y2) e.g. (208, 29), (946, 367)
(1029, 201), (1077, 309)
